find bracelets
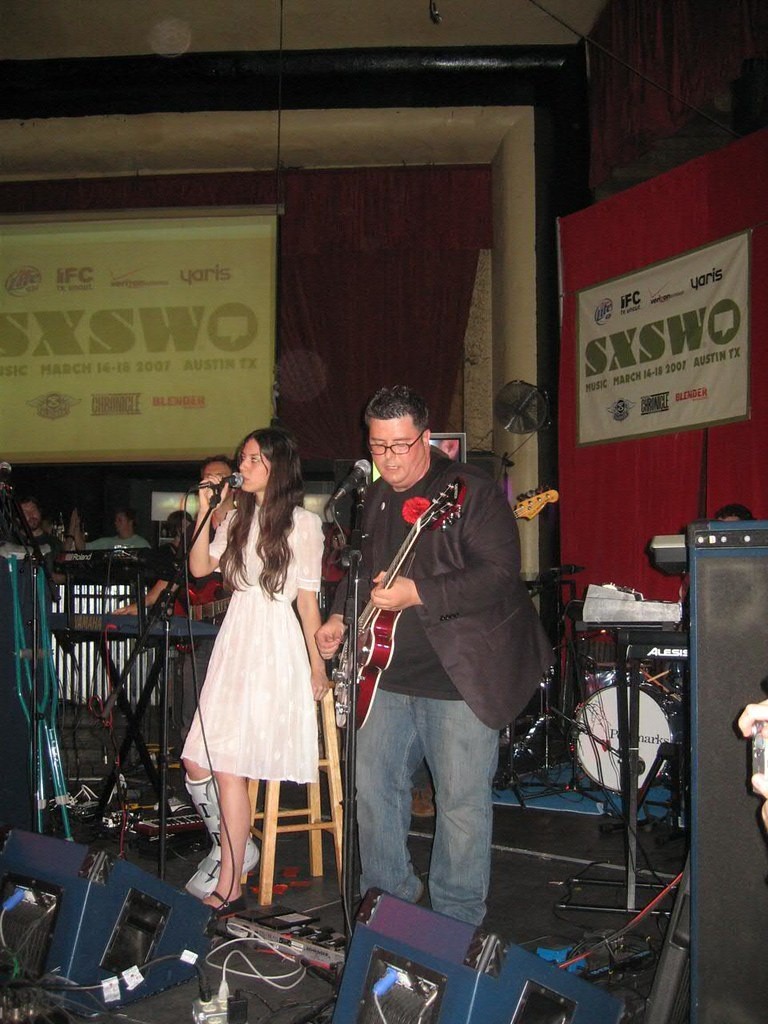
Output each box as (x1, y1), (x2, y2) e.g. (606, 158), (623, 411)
(66, 535), (75, 539)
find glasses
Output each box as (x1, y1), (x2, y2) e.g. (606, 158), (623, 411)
(367, 428), (429, 456)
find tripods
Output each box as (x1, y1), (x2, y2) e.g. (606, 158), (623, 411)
(491, 584), (625, 810)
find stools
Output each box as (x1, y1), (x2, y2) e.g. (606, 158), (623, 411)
(238, 681), (343, 905)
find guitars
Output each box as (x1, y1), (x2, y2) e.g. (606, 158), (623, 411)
(512, 484), (560, 521)
(335, 474), (467, 732)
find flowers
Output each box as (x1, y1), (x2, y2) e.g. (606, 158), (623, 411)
(402, 497), (430, 533)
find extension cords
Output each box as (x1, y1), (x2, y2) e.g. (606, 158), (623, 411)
(192, 992), (248, 1024)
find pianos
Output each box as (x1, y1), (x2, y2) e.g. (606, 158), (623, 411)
(136, 814), (206, 836)
(50, 611), (222, 826)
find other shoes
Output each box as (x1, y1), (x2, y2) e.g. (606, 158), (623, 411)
(154, 798), (185, 812)
(202, 891), (245, 917)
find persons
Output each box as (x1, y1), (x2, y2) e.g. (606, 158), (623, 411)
(19, 496), (151, 579)
(737, 699), (768, 832)
(682, 502), (755, 630)
(181, 427), (330, 915)
(314, 385), (558, 930)
(113, 454), (238, 755)
(167, 510), (193, 548)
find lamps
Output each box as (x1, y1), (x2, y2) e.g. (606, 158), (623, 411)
(144, 479), (201, 521)
(297, 459), (361, 527)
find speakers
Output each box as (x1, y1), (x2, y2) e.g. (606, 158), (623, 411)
(0, 829), (214, 1017)
(331, 887), (625, 1024)
(688, 518), (768, 1024)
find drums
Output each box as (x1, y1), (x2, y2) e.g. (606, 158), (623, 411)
(572, 681), (687, 792)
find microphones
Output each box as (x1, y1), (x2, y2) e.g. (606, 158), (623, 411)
(331, 459), (371, 506)
(0, 462), (11, 490)
(190, 472), (244, 492)
(637, 757), (646, 775)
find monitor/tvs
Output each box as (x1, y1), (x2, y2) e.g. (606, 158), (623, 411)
(372, 432), (467, 484)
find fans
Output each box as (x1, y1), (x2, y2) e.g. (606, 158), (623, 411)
(493, 380), (552, 436)
